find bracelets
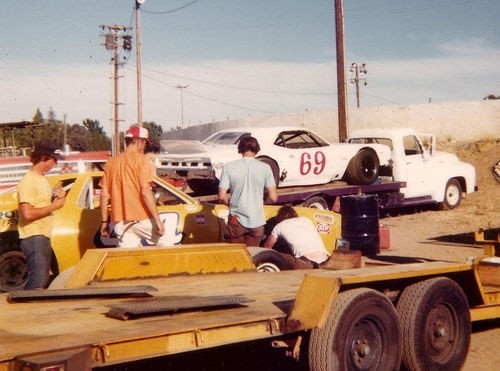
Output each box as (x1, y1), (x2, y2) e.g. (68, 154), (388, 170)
(101, 220), (110, 224)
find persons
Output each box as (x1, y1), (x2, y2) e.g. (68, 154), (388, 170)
(17, 141), (67, 291)
(100, 128), (173, 247)
(265, 205), (331, 269)
(218, 137), (277, 247)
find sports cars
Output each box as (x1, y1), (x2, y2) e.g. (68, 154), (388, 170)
(202, 126), (394, 186)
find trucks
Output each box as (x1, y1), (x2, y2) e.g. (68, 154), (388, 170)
(154, 127), (478, 220)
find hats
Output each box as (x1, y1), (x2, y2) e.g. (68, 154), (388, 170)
(125, 127), (152, 144)
(35, 140), (65, 160)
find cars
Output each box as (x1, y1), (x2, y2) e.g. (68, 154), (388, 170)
(154, 139), (216, 182)
(0, 171), (343, 292)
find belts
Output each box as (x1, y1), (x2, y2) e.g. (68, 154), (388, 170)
(300, 255), (331, 267)
